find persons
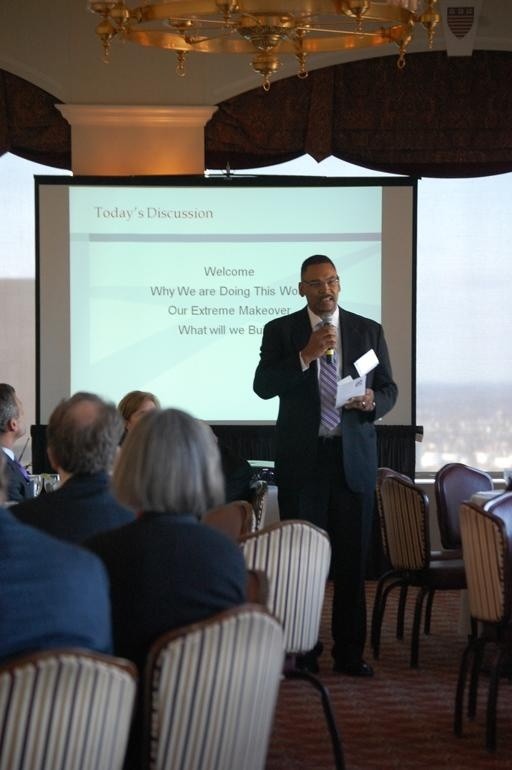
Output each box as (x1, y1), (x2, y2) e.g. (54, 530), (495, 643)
(252, 254), (398, 651)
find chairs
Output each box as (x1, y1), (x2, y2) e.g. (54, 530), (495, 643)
(371, 467), (464, 648)
(486, 493), (512, 541)
(0, 647), (137, 770)
(434, 463), (494, 548)
(202, 501), (255, 540)
(142, 603), (283, 770)
(249, 480), (268, 531)
(452, 502), (512, 751)
(373, 477), (479, 669)
(236, 519), (345, 770)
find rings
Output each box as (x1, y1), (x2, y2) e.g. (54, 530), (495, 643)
(361, 400), (367, 410)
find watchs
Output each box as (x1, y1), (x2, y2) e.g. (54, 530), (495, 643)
(372, 395), (377, 409)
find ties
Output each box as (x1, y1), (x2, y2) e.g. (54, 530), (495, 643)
(14, 459), (31, 482)
(317, 322), (342, 431)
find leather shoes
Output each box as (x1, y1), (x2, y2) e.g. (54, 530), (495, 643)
(333, 658), (375, 678)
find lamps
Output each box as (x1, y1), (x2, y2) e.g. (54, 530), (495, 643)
(88, 1), (439, 92)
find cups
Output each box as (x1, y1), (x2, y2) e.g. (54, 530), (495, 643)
(27, 475), (43, 497)
(44, 474), (60, 493)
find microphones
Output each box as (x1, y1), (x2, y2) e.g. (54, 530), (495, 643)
(319, 310), (335, 364)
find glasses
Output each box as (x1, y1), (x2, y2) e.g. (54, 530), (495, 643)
(302, 277), (338, 287)
